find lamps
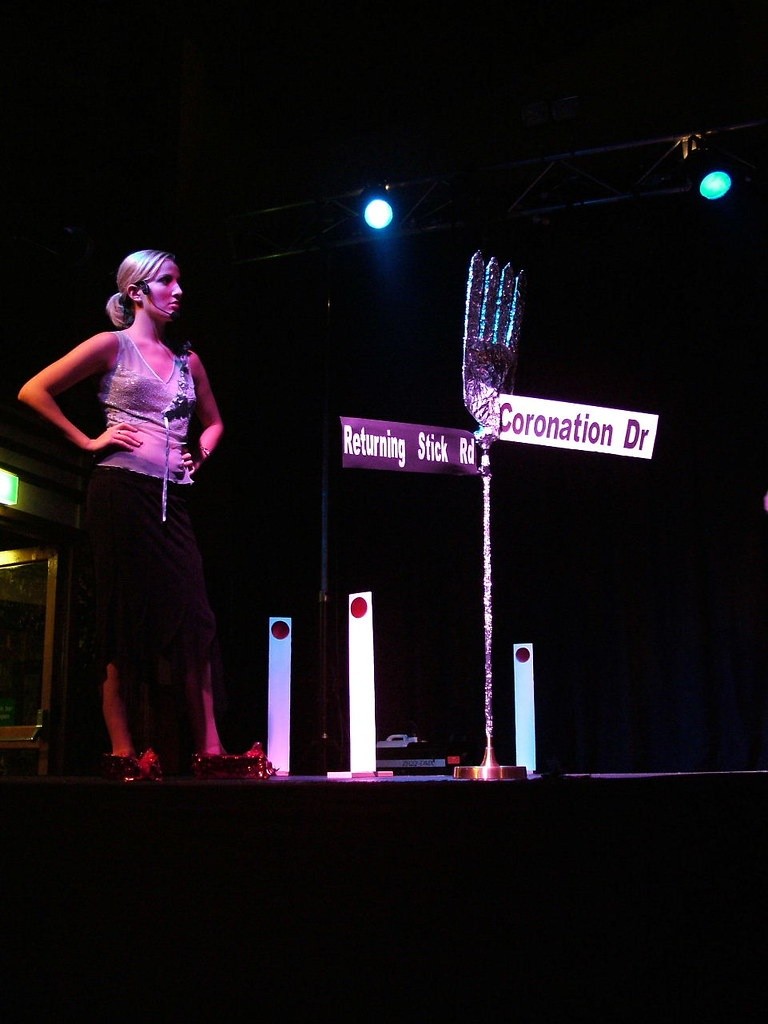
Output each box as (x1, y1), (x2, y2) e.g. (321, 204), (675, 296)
(684, 147), (733, 201)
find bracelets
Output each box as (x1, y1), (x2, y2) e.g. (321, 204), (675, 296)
(200, 445), (210, 457)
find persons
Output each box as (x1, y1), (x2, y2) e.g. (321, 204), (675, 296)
(15, 248), (270, 782)
